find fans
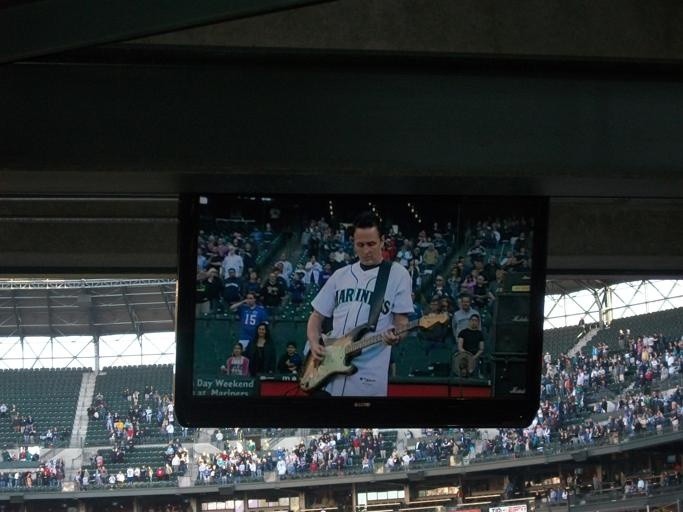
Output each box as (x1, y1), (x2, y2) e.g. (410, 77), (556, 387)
(447, 351), (478, 396)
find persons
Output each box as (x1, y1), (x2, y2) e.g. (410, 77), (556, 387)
(306, 212), (415, 396)
(0, 319), (683, 512)
(193, 202), (531, 394)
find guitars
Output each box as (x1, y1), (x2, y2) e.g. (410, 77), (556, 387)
(301, 313), (451, 393)
